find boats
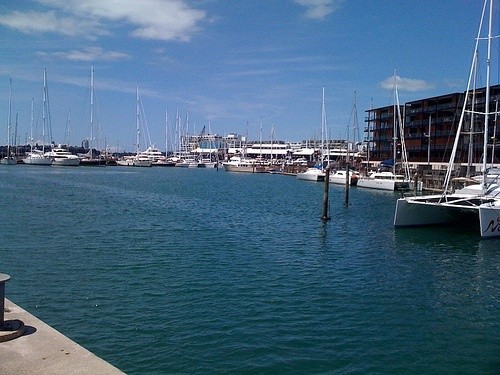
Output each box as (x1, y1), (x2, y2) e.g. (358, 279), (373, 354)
(1, 0), (500, 236)
